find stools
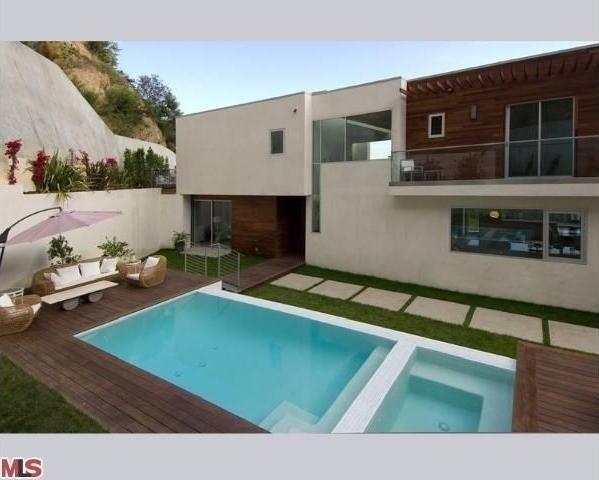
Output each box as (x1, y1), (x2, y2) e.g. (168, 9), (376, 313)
(424, 168), (443, 181)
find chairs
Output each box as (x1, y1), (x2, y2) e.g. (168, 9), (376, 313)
(399, 158), (424, 182)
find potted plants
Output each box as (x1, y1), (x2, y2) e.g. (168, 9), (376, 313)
(171, 230), (192, 251)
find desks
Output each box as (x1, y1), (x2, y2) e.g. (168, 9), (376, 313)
(40, 280), (120, 310)
(119, 259), (141, 275)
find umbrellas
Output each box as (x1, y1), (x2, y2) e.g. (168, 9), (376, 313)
(0, 208), (126, 265)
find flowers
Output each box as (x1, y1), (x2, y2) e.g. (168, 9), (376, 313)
(3, 136), (23, 184)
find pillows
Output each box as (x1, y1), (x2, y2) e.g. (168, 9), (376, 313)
(0, 293), (15, 313)
(144, 257), (160, 269)
(43, 272), (61, 286)
(78, 260), (101, 279)
(55, 264), (82, 283)
(100, 258), (119, 273)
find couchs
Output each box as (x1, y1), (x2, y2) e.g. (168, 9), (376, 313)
(31, 255), (129, 296)
(126, 254), (168, 288)
(0, 293), (44, 336)
